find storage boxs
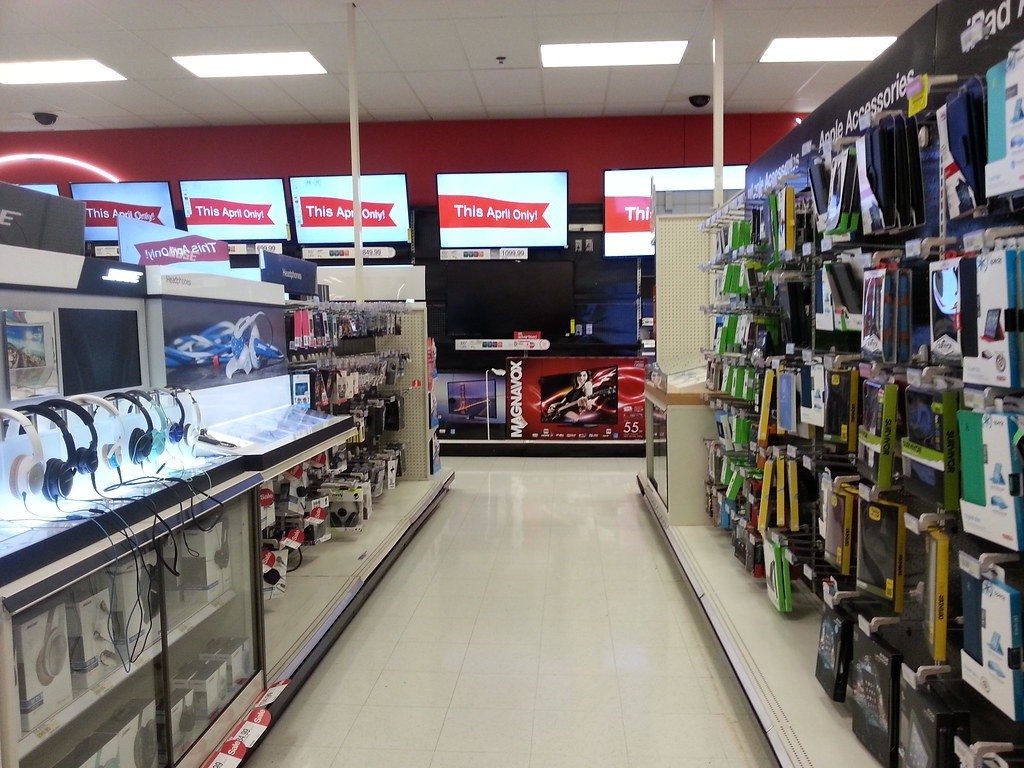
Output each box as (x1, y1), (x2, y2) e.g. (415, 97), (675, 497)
(705, 187), (970, 768)
(12, 519), (250, 768)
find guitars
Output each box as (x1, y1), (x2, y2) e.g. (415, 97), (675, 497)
(547, 386), (618, 420)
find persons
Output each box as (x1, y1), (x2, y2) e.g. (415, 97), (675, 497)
(650, 370), (663, 385)
(547, 370), (593, 420)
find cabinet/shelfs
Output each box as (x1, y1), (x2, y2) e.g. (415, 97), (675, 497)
(375, 302), (455, 488)
(643, 214), (718, 526)
(0, 473), (266, 768)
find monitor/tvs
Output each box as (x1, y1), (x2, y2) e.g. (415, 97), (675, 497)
(539, 365), (618, 428)
(179, 178), (293, 244)
(445, 263), (574, 337)
(435, 169), (570, 250)
(603, 164), (751, 261)
(288, 173), (412, 246)
(68, 180), (177, 244)
(447, 379), (497, 422)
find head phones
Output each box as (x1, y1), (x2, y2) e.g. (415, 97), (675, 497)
(179, 688), (196, 732)
(215, 518), (230, 569)
(36, 605), (68, 686)
(134, 708), (157, 768)
(1, 384), (202, 501)
(329, 501), (360, 527)
(225, 311), (285, 379)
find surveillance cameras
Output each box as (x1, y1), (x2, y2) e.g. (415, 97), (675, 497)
(33, 113), (58, 125)
(689, 95), (711, 108)
